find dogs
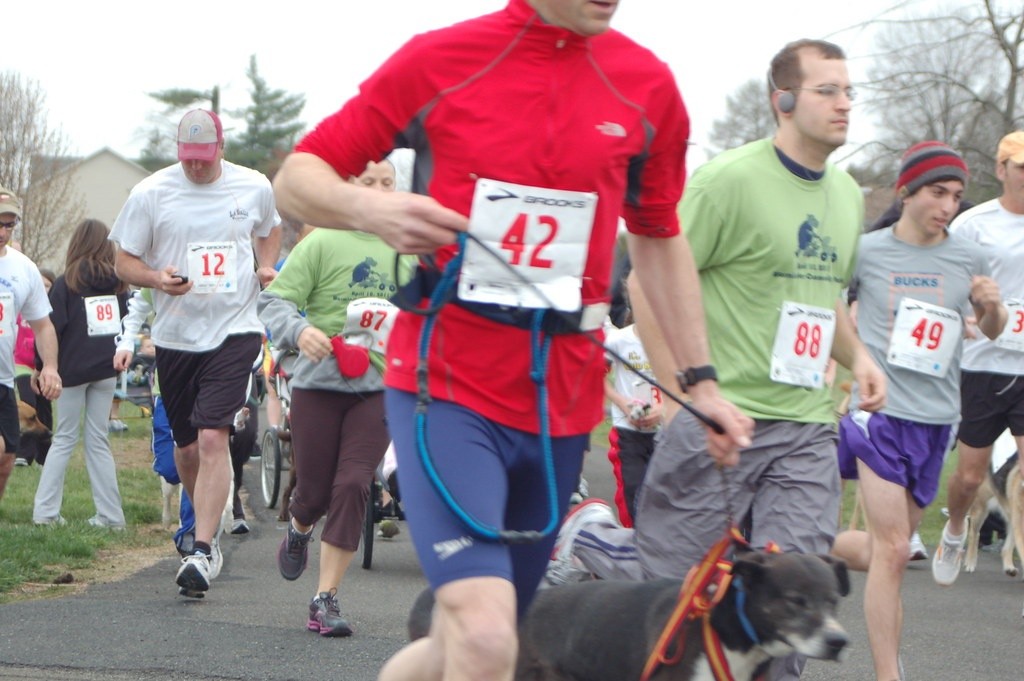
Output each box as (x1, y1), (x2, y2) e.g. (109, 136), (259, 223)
(406, 548), (853, 681)
(15, 401), (52, 437)
(963, 451), (1024, 578)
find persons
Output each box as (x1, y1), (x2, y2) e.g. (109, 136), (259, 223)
(844, 141), (1009, 680)
(850, 192), (977, 563)
(109, 369), (130, 431)
(931, 131), (1023, 588)
(30, 218), (135, 531)
(0, 187), (63, 507)
(270, 1), (756, 680)
(14, 268), (57, 465)
(601, 304), (666, 529)
(627, 37), (889, 680)
(256, 158), (421, 639)
(106, 106), (283, 600)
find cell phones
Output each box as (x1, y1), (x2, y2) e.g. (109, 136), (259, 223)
(171, 274), (188, 284)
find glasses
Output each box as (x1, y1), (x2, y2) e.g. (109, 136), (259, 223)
(783, 84), (855, 102)
(0, 220), (18, 228)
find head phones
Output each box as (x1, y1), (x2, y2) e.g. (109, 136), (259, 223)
(769, 68), (795, 113)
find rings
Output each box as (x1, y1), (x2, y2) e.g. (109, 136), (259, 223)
(56, 384), (59, 387)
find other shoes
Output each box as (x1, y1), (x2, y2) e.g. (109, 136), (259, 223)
(34, 513), (68, 527)
(13, 457), (30, 467)
(108, 418), (128, 432)
(85, 515), (127, 532)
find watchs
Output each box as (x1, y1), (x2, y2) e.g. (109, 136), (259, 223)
(675, 365), (720, 391)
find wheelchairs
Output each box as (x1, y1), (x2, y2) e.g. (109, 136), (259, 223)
(261, 348), (376, 570)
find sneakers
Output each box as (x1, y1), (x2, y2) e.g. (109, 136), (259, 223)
(206, 510), (224, 581)
(229, 517), (250, 534)
(173, 551), (211, 598)
(930, 516), (968, 587)
(907, 531), (929, 560)
(306, 590), (353, 638)
(533, 500), (619, 614)
(277, 514), (315, 582)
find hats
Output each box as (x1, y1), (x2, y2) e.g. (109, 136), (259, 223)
(175, 109), (224, 163)
(997, 131), (1024, 164)
(895, 140), (968, 199)
(0, 189), (22, 220)
(36, 268), (57, 286)
